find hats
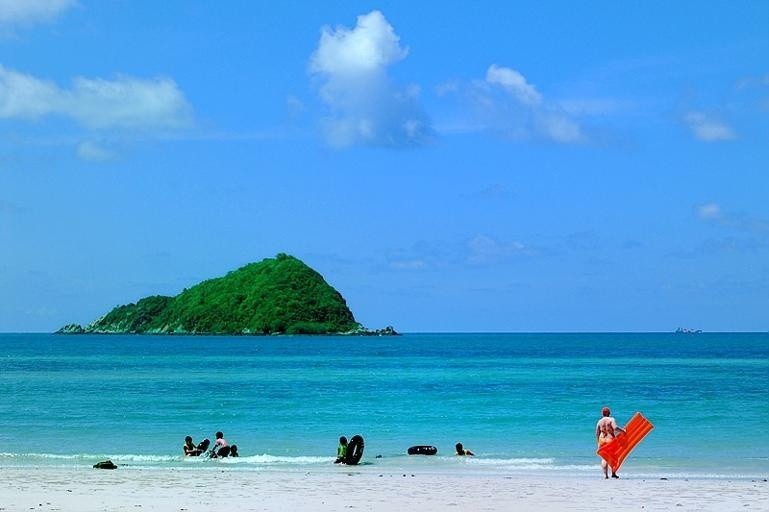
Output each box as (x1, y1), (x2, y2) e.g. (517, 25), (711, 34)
(602, 407), (610, 415)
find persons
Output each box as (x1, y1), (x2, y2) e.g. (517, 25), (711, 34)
(209, 432), (230, 459)
(334, 435), (350, 462)
(181, 435), (204, 458)
(596, 408), (628, 480)
(452, 442), (476, 457)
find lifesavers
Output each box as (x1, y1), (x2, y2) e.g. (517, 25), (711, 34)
(408, 445), (436, 455)
(345, 435), (364, 464)
(194, 439), (210, 454)
